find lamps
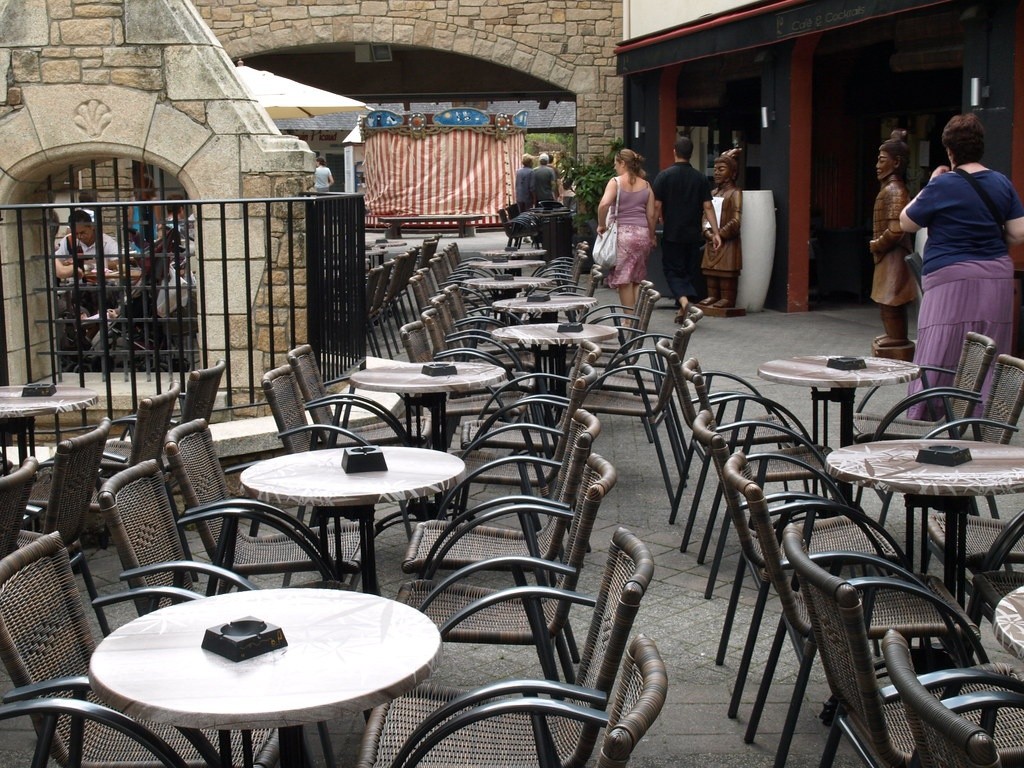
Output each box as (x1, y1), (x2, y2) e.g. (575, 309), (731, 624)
(372, 44), (393, 62)
(354, 44), (372, 62)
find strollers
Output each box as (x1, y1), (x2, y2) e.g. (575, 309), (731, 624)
(54, 226), (192, 372)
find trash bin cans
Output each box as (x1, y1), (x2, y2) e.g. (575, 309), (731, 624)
(539, 211), (573, 265)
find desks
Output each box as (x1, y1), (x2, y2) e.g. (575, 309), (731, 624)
(0, 383), (98, 469)
(468, 257), (546, 296)
(87, 588), (444, 768)
(992, 585), (1024, 661)
(491, 322), (620, 427)
(240, 445), (467, 598)
(365, 249), (387, 257)
(365, 239), (407, 265)
(755, 354), (922, 519)
(463, 274), (552, 326)
(491, 296), (598, 393)
(824, 439), (1024, 675)
(349, 361), (507, 524)
(481, 248), (547, 292)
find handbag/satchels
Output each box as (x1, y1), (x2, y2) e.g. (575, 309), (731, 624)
(592, 221), (618, 270)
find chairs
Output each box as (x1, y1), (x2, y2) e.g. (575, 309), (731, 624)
(0, 201), (1024, 768)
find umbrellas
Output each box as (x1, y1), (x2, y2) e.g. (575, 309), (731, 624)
(235, 56), (367, 122)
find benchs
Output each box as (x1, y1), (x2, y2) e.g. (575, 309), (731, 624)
(378, 215), (486, 239)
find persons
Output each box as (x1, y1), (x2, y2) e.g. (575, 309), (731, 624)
(869, 129), (918, 347)
(47, 173), (196, 373)
(700, 148), (746, 310)
(596, 137), (722, 330)
(515, 152), (564, 214)
(899, 114), (1024, 422)
(315, 157), (334, 192)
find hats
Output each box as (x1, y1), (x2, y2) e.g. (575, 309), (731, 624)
(539, 154), (549, 163)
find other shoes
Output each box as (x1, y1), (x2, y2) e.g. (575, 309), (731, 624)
(676, 303), (694, 326)
